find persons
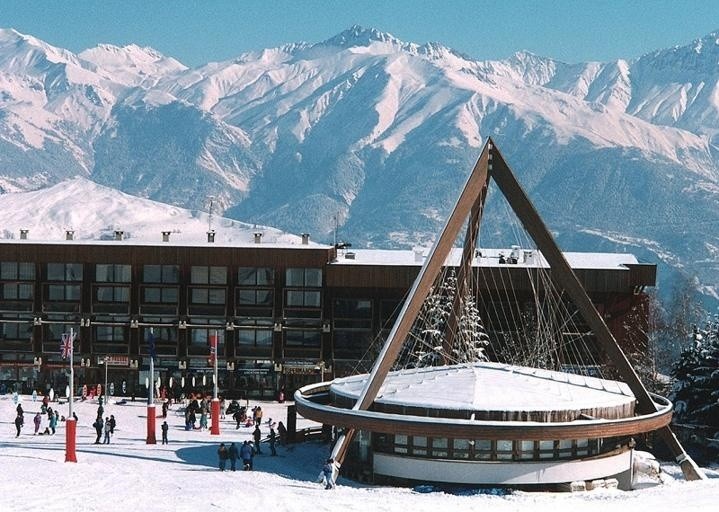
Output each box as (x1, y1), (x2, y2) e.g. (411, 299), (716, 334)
(326, 458), (339, 489)
(216, 442), (228, 469)
(323, 459), (331, 491)
(0, 376), (290, 457)
(247, 441), (255, 469)
(227, 442), (240, 472)
(239, 441), (252, 471)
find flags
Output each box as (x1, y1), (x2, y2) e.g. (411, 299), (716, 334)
(57, 332), (77, 360)
(147, 331), (156, 361)
(207, 335), (220, 367)
